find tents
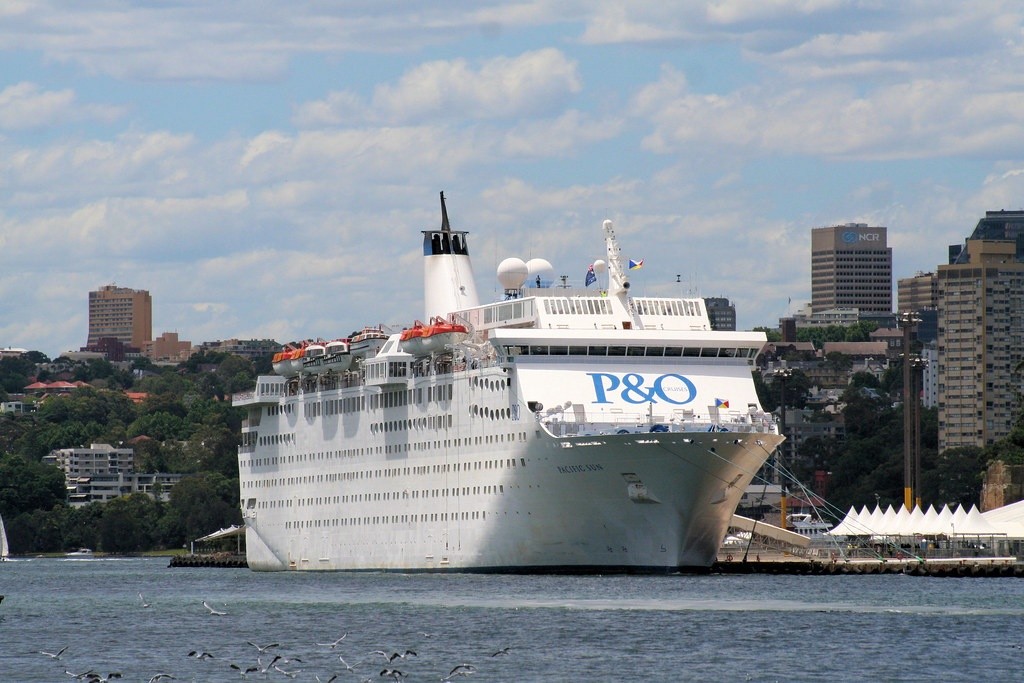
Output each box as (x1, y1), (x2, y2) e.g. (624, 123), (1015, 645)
(824, 503), (1008, 558)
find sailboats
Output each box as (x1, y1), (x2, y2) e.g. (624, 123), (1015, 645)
(0, 515), (9, 561)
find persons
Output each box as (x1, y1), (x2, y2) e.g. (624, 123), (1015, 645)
(888, 545), (893, 558)
(974, 547), (979, 557)
(535, 274), (541, 288)
(876, 544), (882, 559)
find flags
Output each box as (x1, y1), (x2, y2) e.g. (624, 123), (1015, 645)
(586, 264), (596, 287)
(629, 260), (643, 270)
(716, 398), (729, 408)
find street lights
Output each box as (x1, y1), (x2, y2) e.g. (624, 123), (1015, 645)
(898, 312), (923, 510)
(899, 353), (928, 512)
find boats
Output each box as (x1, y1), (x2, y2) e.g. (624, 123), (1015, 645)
(725, 498), (849, 546)
(231, 190), (810, 572)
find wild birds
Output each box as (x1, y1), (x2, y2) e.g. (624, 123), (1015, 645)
(314, 633), (347, 648)
(202, 600), (227, 616)
(422, 633), (433, 638)
(315, 650), (477, 683)
(230, 641), (307, 680)
(188, 651), (214, 661)
(65, 669), (125, 683)
(491, 647), (510, 657)
(148, 674), (176, 683)
(41, 646), (68, 660)
(139, 594), (152, 607)
